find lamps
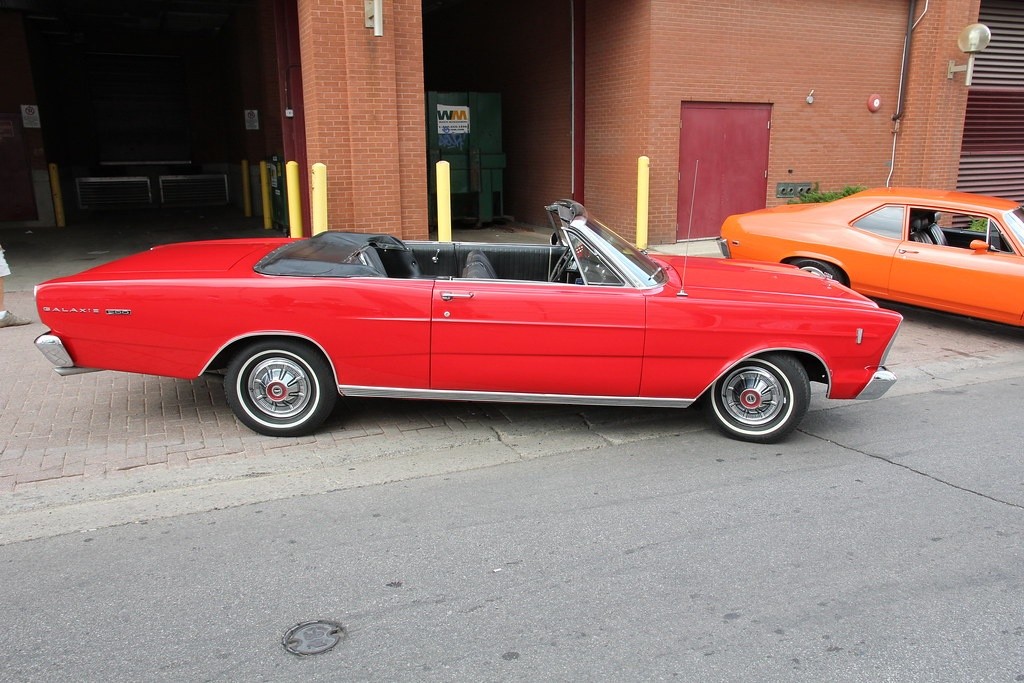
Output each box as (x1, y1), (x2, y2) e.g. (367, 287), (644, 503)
(947, 23), (991, 87)
(364, 0), (384, 38)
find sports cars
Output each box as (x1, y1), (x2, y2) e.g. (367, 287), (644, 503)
(714, 186), (1024, 331)
(29, 198), (905, 443)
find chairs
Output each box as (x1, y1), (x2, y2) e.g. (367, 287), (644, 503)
(910, 216), (933, 244)
(462, 261), (494, 279)
(927, 212), (948, 245)
(466, 247), (497, 279)
(359, 246), (388, 277)
